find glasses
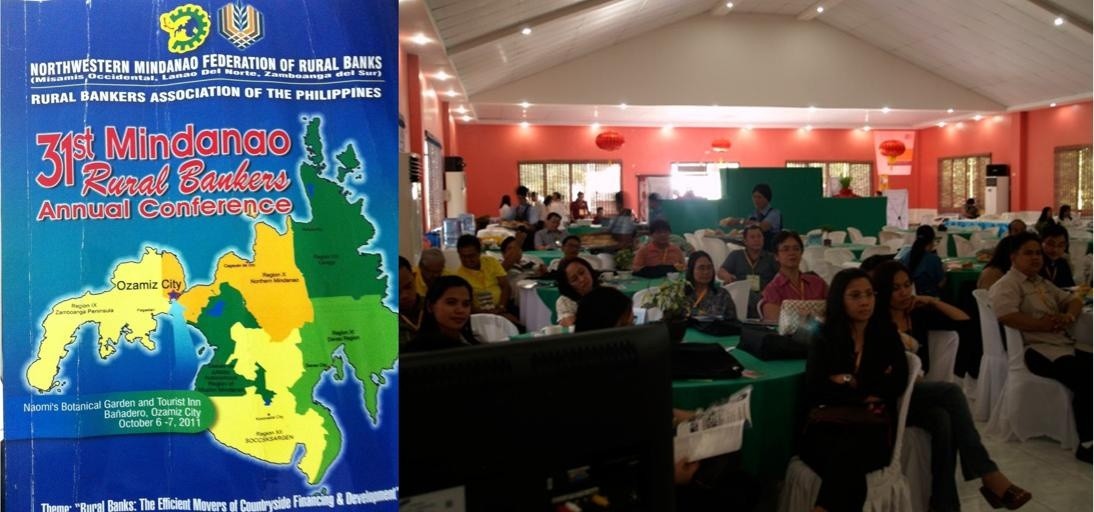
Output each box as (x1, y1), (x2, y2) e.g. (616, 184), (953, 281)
(845, 291), (876, 299)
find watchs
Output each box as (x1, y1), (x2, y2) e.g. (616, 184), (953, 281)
(843, 371), (852, 388)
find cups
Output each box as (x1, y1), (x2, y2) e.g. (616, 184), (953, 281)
(600, 272), (614, 281)
(666, 272), (680, 280)
(539, 325), (561, 335)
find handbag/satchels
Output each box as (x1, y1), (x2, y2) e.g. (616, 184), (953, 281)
(779, 300), (827, 335)
(671, 342), (744, 378)
(736, 328), (816, 360)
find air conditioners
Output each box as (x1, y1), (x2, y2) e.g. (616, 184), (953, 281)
(984, 175), (1009, 215)
(398, 152), (422, 266)
(445, 170), (467, 219)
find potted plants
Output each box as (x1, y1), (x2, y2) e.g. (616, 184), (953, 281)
(840, 176), (852, 194)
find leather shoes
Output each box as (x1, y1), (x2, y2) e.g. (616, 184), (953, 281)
(1075, 442), (1093, 463)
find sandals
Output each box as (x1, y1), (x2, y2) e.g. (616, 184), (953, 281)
(981, 486), (1031, 509)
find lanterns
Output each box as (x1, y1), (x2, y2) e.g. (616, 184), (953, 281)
(711, 140), (732, 152)
(595, 132), (626, 152)
(878, 139), (906, 163)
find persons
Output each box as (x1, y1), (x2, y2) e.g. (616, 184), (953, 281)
(402, 184), (1093, 341)
(790, 267), (908, 512)
(988, 232), (1093, 465)
(522, 285), (676, 511)
(860, 260), (1032, 512)
